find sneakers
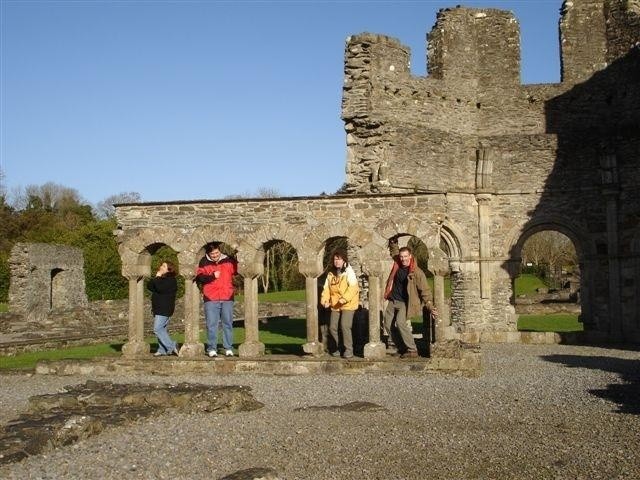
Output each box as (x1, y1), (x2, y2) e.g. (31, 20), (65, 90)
(208, 350), (218, 357)
(152, 350), (164, 357)
(409, 349), (418, 358)
(172, 343), (180, 358)
(331, 348), (354, 359)
(225, 349), (233, 357)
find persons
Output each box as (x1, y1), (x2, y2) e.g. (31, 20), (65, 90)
(146, 260), (180, 357)
(320, 251), (361, 358)
(382, 237), (441, 359)
(195, 244), (237, 357)
